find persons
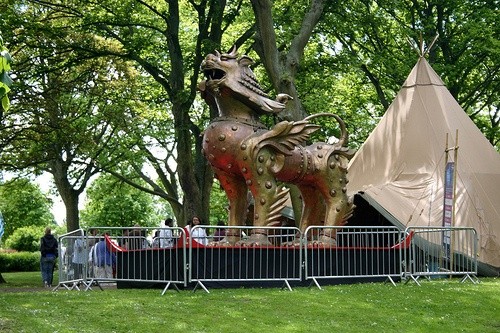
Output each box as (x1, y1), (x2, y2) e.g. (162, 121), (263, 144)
(71, 228), (118, 281)
(122, 215), (208, 249)
(39, 227), (58, 288)
(214, 221), (226, 241)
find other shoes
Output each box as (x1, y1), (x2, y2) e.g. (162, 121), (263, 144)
(49, 285), (51, 287)
(44, 281), (48, 288)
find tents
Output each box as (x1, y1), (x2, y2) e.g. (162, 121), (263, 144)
(280, 57), (500, 276)
